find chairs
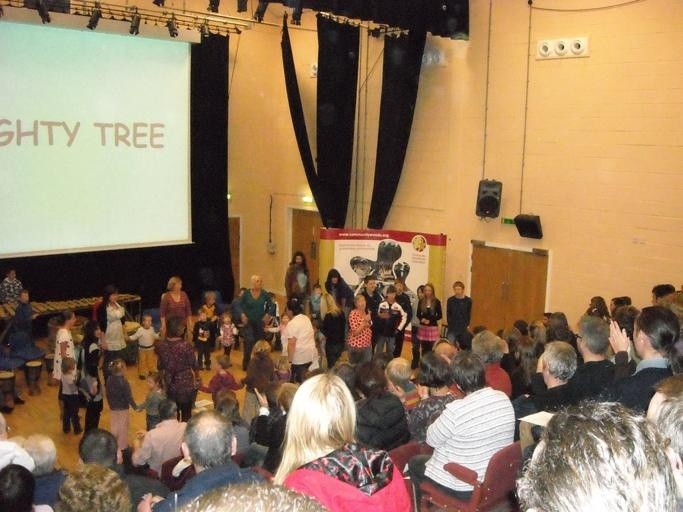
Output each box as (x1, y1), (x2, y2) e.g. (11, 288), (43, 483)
(388, 439), (523, 511)
(143, 456), (195, 489)
(142, 307), (161, 332)
(0, 331), (45, 369)
(231, 454), (275, 482)
(200, 290), (224, 314)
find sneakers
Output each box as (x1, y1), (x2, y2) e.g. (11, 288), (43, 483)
(139, 375), (146, 381)
(199, 365), (205, 370)
(206, 364), (211, 370)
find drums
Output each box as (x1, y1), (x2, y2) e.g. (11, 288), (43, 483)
(45, 355), (56, 385)
(26, 361), (42, 396)
(124, 322), (140, 366)
(1, 371), (15, 413)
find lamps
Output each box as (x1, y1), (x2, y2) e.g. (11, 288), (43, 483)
(236, 27), (241, 34)
(290, 5), (302, 26)
(167, 19), (178, 36)
(152, 1), (165, 7)
(254, 2), (268, 23)
(129, 15), (141, 36)
(200, 27), (207, 36)
(207, 1), (220, 14)
(36, 2), (51, 24)
(237, 1), (248, 13)
(87, 10), (101, 30)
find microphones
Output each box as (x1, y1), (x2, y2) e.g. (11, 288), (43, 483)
(365, 309), (371, 324)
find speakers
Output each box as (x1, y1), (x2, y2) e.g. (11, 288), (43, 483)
(476, 180), (502, 217)
(514, 215), (542, 238)
